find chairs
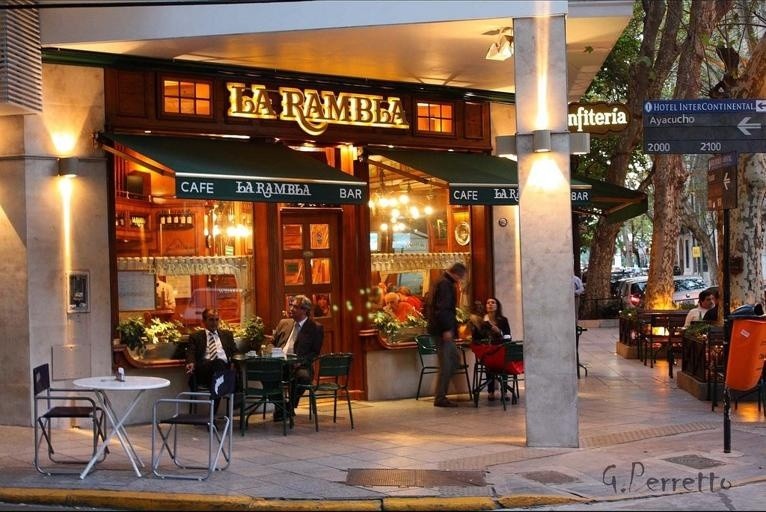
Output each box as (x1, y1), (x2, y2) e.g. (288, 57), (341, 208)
(241, 358), (292, 437)
(33, 363), (110, 479)
(476, 337), (527, 413)
(576, 324), (588, 379)
(150, 368), (237, 481)
(639, 309), (739, 382)
(293, 350), (355, 433)
(183, 347), (242, 418)
(413, 332), (475, 400)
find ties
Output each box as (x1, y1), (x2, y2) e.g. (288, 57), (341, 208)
(285, 322), (300, 357)
(209, 331), (217, 361)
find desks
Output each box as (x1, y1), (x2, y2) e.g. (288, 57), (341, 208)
(460, 338), (522, 360)
(71, 375), (172, 481)
(229, 353), (300, 365)
(579, 325), (587, 336)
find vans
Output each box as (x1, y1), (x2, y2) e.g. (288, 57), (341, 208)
(182, 286), (241, 328)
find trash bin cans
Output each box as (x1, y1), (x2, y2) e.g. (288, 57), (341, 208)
(724, 303), (766, 390)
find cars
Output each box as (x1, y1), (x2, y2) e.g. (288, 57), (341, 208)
(580, 264), (711, 310)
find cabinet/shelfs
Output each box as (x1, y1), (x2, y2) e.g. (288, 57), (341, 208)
(114, 201), (152, 241)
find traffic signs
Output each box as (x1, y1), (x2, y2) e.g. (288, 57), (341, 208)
(643, 99), (766, 154)
(706, 152), (738, 211)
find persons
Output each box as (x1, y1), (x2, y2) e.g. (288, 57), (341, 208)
(469, 295), (512, 402)
(153, 279), (176, 310)
(258, 293), (325, 423)
(683, 288), (716, 329)
(359, 280), (426, 330)
(183, 307), (241, 431)
(702, 286), (719, 321)
(426, 261), (468, 407)
(573, 274), (582, 322)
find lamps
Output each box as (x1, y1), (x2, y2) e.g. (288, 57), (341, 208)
(57, 156), (83, 178)
(570, 132), (590, 156)
(484, 35), (514, 63)
(532, 129), (551, 153)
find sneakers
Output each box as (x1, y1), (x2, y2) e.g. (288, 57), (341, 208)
(433, 399), (458, 407)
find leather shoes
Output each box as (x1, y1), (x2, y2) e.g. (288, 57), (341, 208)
(487, 395), (511, 401)
(273, 403), (296, 422)
